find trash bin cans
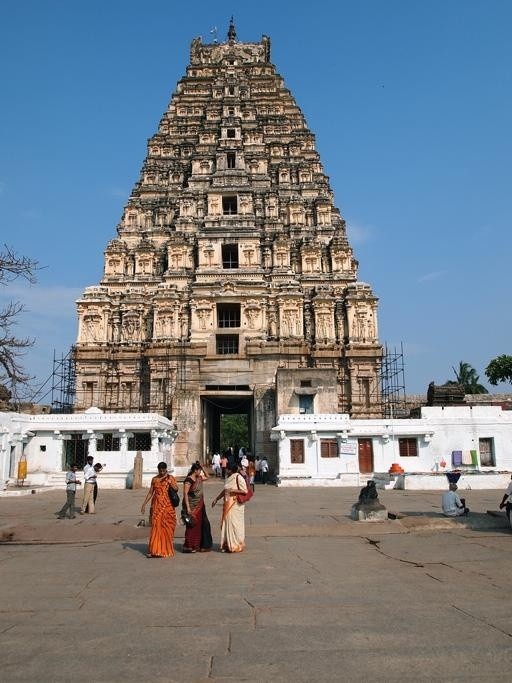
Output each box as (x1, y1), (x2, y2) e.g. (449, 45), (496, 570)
(452, 449), (478, 466)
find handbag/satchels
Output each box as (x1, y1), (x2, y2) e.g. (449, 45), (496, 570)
(235, 474), (254, 504)
(167, 475), (181, 509)
(179, 511), (197, 527)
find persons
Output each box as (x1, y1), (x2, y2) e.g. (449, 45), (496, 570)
(80, 462), (102, 513)
(442, 482), (469, 516)
(81, 308), (370, 341)
(83, 455), (107, 511)
(211, 445), (269, 486)
(212, 462), (248, 552)
(183, 462), (213, 552)
(55, 463), (82, 518)
(141, 461), (178, 556)
(500, 474), (511, 521)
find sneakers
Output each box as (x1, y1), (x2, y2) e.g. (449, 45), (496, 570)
(55, 507), (98, 521)
(147, 544), (246, 559)
